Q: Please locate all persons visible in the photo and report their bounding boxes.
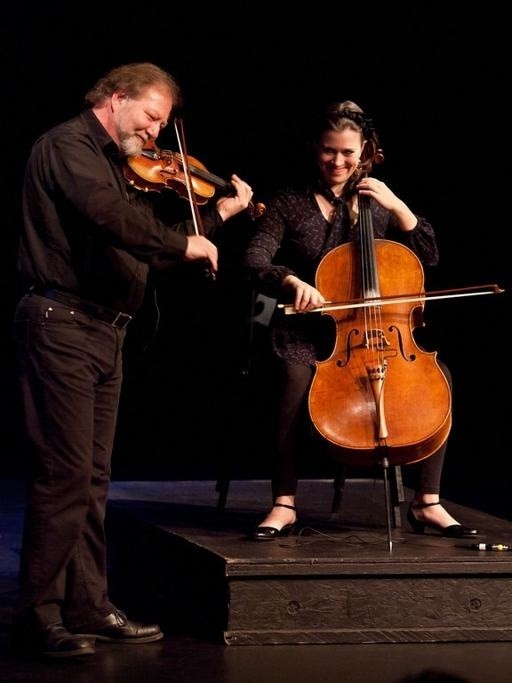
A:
[244,98,483,542]
[13,60,255,661]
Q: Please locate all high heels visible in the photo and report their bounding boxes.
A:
[406,501,479,538]
[253,502,299,540]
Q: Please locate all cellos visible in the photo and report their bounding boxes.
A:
[305,139,454,463]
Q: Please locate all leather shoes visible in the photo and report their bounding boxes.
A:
[42,622,96,658]
[73,608,165,644]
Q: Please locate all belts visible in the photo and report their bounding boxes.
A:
[34,287,134,330]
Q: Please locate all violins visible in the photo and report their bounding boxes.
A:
[123,142,266,219]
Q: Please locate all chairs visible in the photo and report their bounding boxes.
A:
[214,289,405,527]
